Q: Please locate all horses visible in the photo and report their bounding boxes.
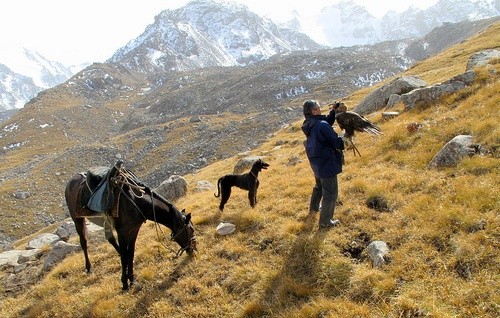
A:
[65,161,197,291]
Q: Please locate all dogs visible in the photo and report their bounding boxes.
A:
[214,159,270,212]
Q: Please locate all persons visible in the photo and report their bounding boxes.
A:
[301,100,353,229]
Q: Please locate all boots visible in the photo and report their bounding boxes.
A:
[310,187,322,212]
[318,196,340,230]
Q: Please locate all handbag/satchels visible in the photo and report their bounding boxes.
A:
[87,174,114,213]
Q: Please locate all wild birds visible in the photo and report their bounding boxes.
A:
[329,101,386,159]
